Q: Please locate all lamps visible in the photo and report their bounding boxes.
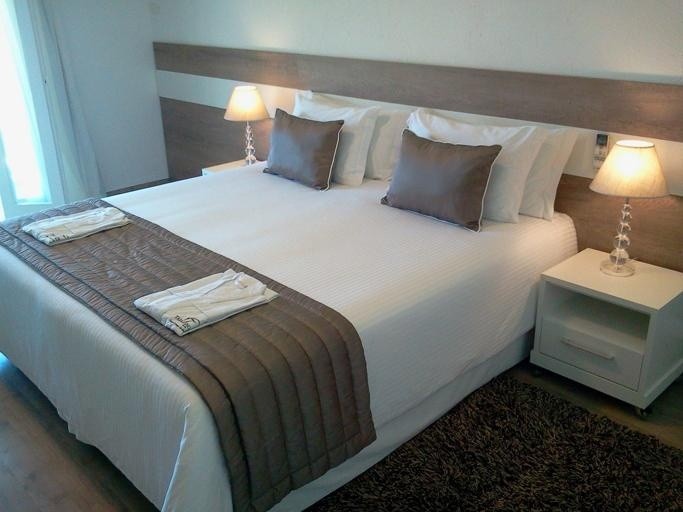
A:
[586,136,672,278]
[223,84,270,165]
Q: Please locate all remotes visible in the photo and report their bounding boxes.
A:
[593,133,609,169]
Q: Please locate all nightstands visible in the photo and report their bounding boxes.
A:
[528,245,683,420]
[201,158,262,174]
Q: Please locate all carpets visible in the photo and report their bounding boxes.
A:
[304,372,682,510]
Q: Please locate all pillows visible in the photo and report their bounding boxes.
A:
[516,123,580,220]
[383,108,549,227]
[302,87,413,182]
[262,107,345,192]
[291,91,381,187]
[381,128,503,234]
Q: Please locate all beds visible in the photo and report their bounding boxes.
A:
[0,158,579,510]
